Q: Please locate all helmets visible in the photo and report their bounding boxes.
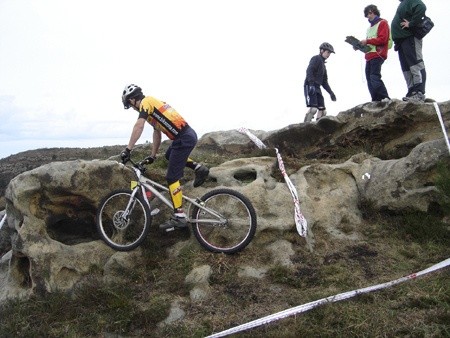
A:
[319,42,335,53]
[122,83,141,109]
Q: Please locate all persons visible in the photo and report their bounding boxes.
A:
[304,42,336,123]
[353,5,393,101]
[120,83,210,227]
[391,0,427,102]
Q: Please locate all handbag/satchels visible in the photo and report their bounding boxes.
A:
[411,14,434,40]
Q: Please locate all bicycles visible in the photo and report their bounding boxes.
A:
[94,152,257,255]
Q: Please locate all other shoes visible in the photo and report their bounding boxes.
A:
[193,165,209,187]
[159,215,188,230]
[402,91,426,102]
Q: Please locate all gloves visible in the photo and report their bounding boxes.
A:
[120,148,131,164]
[144,156,155,164]
[330,93,336,101]
